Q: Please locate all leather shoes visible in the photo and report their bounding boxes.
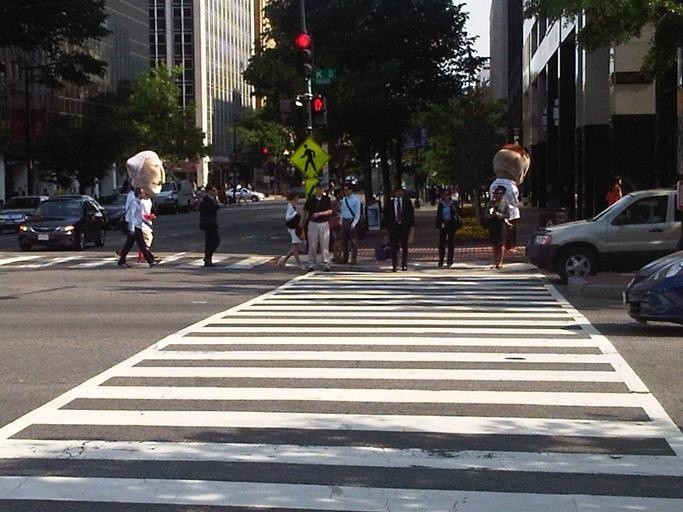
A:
[277,258,503,272]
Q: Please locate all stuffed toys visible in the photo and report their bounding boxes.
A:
[489,143,531,251]
[125,149,166,261]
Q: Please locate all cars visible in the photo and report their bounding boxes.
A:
[0,178,266,250]
[622,249,681,330]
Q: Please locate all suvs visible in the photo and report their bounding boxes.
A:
[527,187,681,285]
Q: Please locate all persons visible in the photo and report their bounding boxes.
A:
[171,183,252,214]
[487,185,510,268]
[278,184,363,269]
[199,186,221,267]
[363,184,483,271]
[17,185,64,196]
[605,173,623,206]
[117,187,162,268]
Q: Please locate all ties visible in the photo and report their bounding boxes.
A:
[395,198,403,226]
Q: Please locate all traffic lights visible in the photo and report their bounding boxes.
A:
[310,94,328,127]
[262,147,274,155]
[292,30,314,78]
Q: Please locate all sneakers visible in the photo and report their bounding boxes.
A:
[118,256,161,270]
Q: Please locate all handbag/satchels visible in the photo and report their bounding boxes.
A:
[116,216,131,235]
[286,212,301,230]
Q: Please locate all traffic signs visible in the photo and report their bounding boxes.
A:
[311,66,337,85]
